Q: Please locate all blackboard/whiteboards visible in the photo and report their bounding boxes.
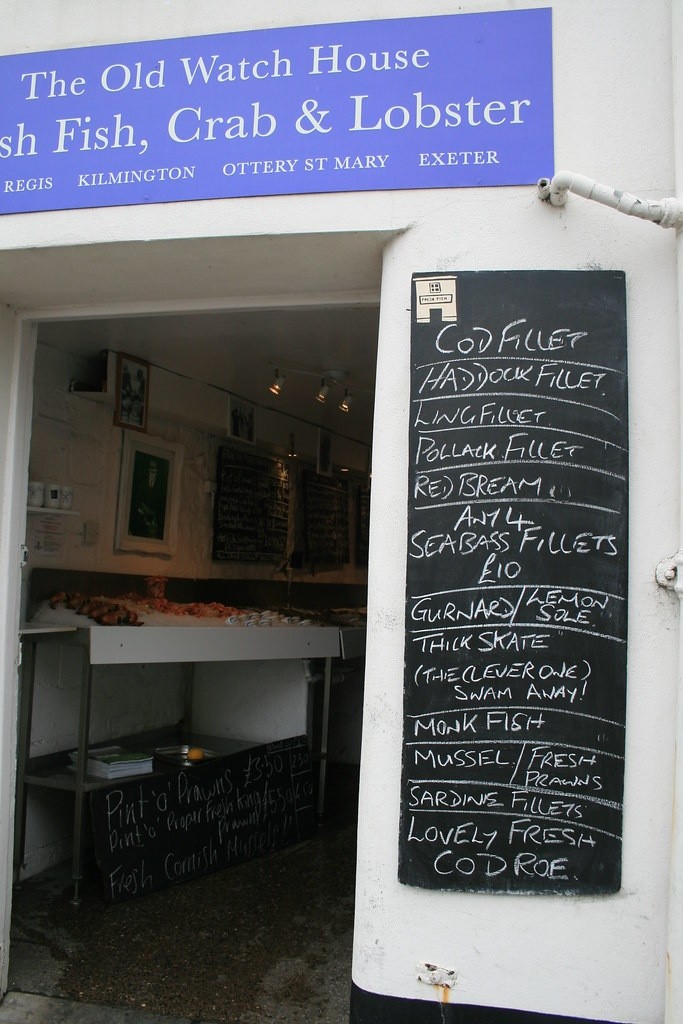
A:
[90,734,317,905]
[355,485,371,569]
[397,268,630,895]
[212,445,289,566]
[302,469,351,565]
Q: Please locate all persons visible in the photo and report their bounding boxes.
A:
[231,407,253,441]
[131,458,163,540]
[120,364,145,401]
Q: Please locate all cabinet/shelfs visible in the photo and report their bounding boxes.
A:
[15,627,333,906]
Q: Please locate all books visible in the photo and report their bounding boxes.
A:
[70,746,154,780]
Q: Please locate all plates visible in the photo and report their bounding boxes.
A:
[152,743,221,766]
[69,745,154,780]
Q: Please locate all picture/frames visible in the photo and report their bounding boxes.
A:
[317,427,334,477]
[227,396,257,444]
[115,428,185,556]
[113,352,150,434]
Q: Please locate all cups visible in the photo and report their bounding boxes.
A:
[28,481,45,507]
[42,484,60,509]
[59,486,73,510]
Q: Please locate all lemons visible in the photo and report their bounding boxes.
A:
[188,749,203,759]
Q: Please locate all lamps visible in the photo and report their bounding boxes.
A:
[269,370,289,395]
[320,369,346,382]
[315,377,330,403]
[338,388,352,412]
